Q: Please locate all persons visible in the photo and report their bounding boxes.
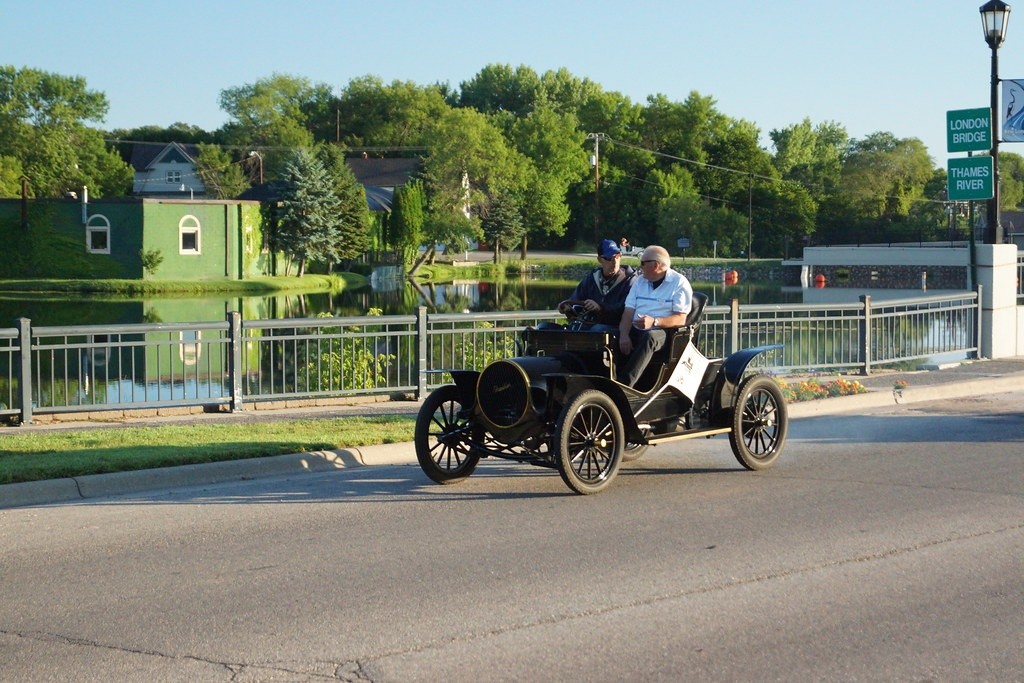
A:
[622,238,632,252]
[589,246,693,388]
[535,240,636,331]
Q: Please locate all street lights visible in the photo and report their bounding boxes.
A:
[980,0,1013,244]
[248,150,264,186]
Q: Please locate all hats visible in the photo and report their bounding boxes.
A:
[597,239,620,257]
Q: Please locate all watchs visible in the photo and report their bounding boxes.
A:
[654,317,659,327]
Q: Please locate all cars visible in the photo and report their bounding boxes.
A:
[413,289,790,497]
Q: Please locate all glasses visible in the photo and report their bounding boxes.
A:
[600,253,620,260]
[641,259,660,266]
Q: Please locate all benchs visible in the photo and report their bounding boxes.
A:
[651,291,709,363]
